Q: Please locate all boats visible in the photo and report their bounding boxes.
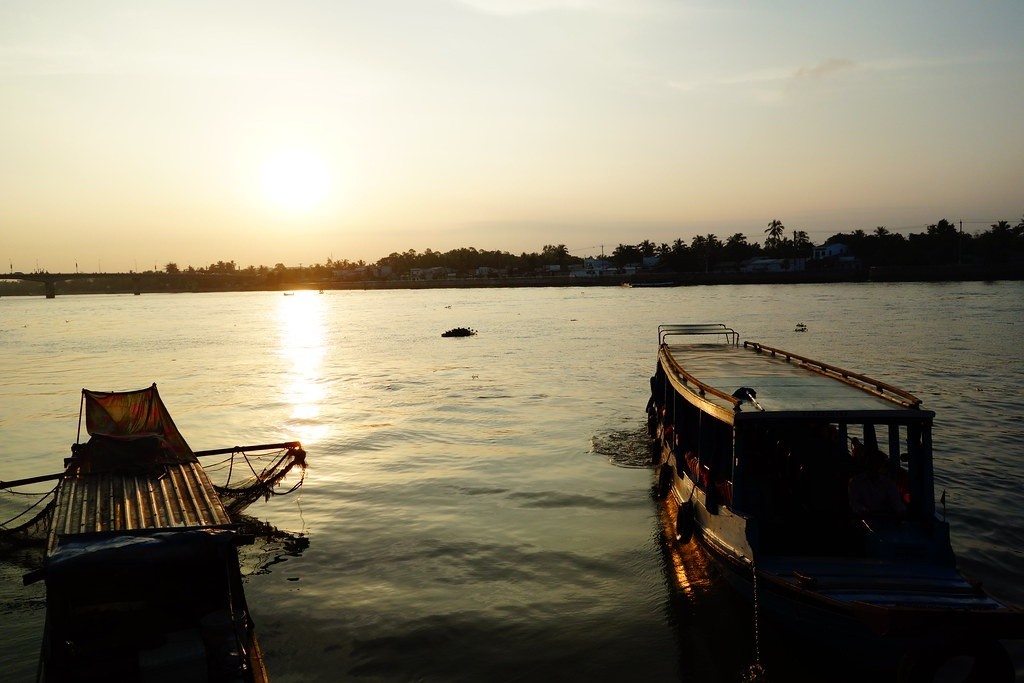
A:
[33,434,270,683]
[647,323,1024,671]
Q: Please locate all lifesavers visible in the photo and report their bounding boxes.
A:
[652,438,661,465]
[675,502,694,544]
[648,414,656,436]
[659,462,669,497]
